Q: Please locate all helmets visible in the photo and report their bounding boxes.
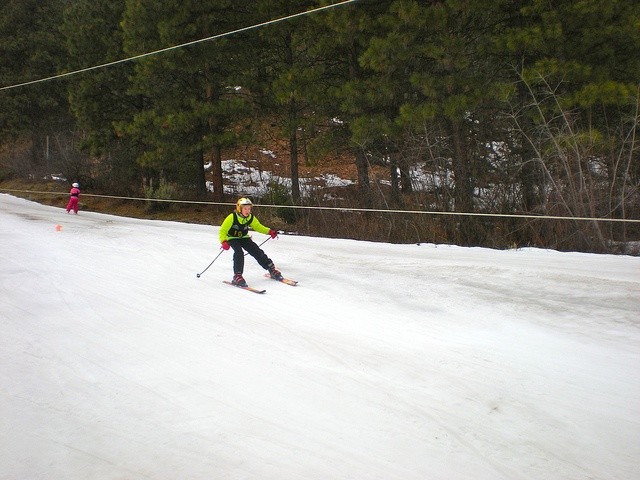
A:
[72,183,79,188]
[236,197,253,213]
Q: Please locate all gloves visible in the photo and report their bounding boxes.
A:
[222,240,230,250]
[269,229,277,239]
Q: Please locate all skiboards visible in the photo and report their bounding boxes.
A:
[222,272,299,294]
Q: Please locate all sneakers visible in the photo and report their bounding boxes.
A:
[232,272,248,287]
[269,264,284,281]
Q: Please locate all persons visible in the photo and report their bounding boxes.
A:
[218,197,282,285]
[65,183,81,215]
